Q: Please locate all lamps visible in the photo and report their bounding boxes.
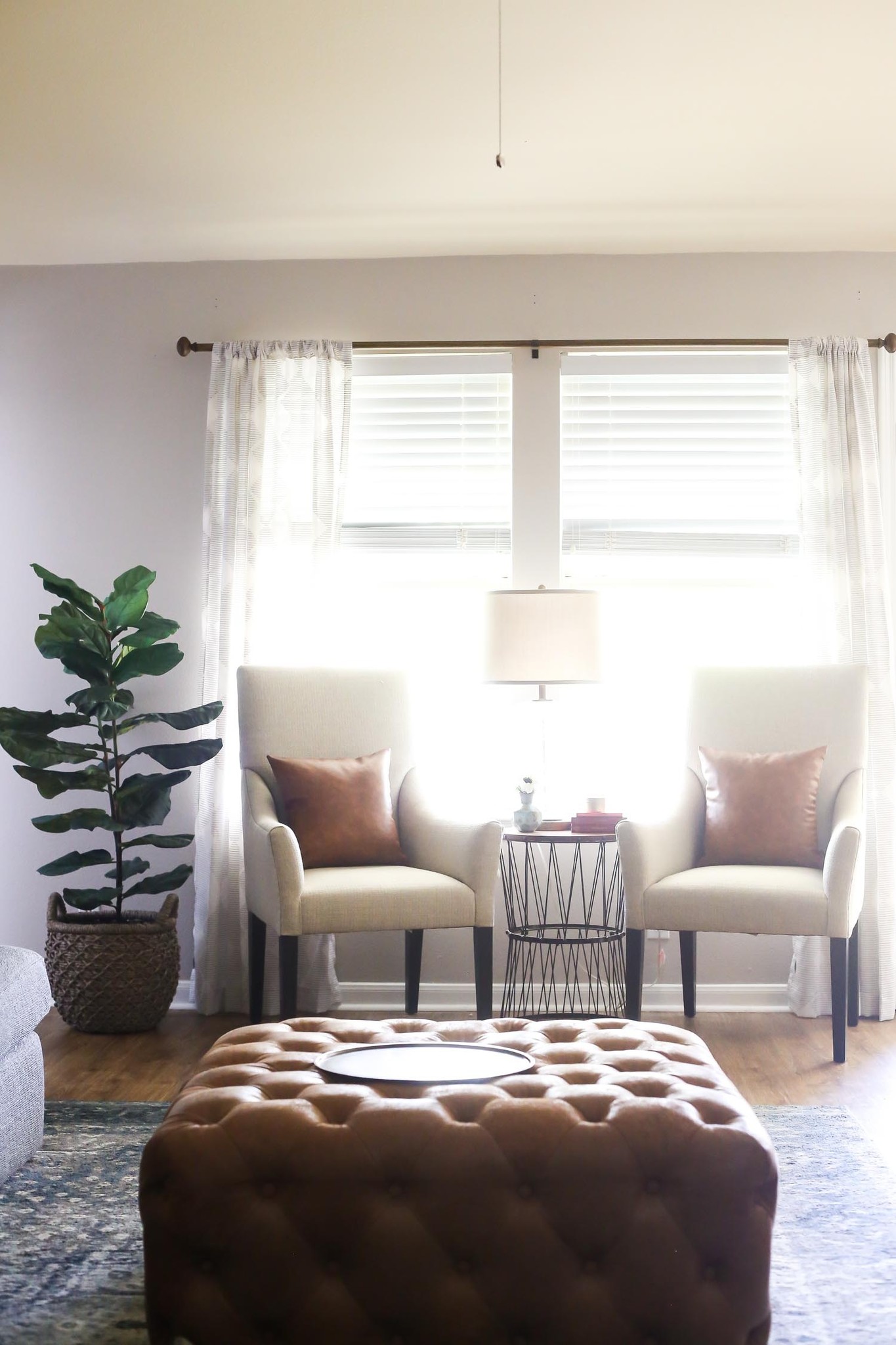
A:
[487,585,601,830]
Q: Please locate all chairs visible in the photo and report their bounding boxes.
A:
[615,661,865,1063]
[237,666,504,1025]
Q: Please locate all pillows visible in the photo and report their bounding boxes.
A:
[697,746,828,870]
[266,749,409,869]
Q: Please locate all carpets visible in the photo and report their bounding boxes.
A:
[0,1100,896,1345]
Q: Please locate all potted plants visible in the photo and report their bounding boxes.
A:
[0,564,223,1032]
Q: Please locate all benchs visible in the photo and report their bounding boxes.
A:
[138,1016,778,1345]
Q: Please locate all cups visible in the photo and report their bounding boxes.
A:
[587,798,605,812]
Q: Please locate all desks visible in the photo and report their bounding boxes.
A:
[500,827,626,1018]
